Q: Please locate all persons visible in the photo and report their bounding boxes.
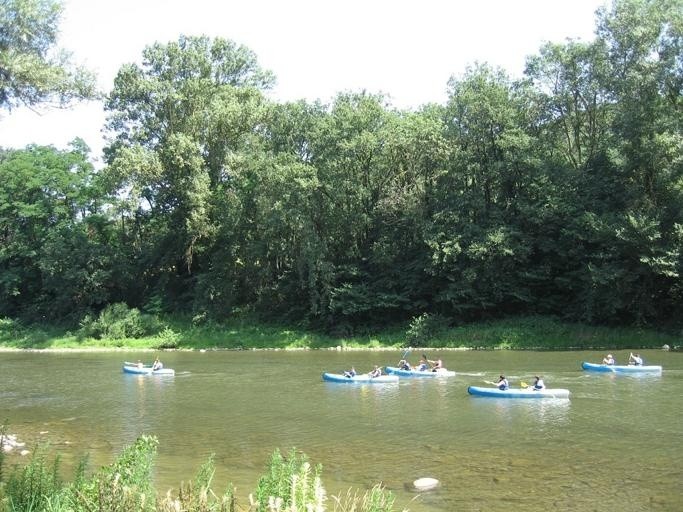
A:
[491,375,508,391]
[398,360,411,371]
[344,367,356,378]
[368,366,381,378]
[133,360,143,369]
[431,362,441,372]
[604,354,614,366]
[152,360,162,371]
[530,376,545,391]
[631,352,642,366]
[416,354,428,372]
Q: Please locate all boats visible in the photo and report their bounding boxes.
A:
[468,386,569,397]
[582,363,662,373]
[123,366,175,375]
[322,367,455,383]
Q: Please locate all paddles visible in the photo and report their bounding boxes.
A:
[485,381,497,385]
[125,361,147,366]
[423,354,437,372]
[147,356,159,375]
[521,382,541,390]
